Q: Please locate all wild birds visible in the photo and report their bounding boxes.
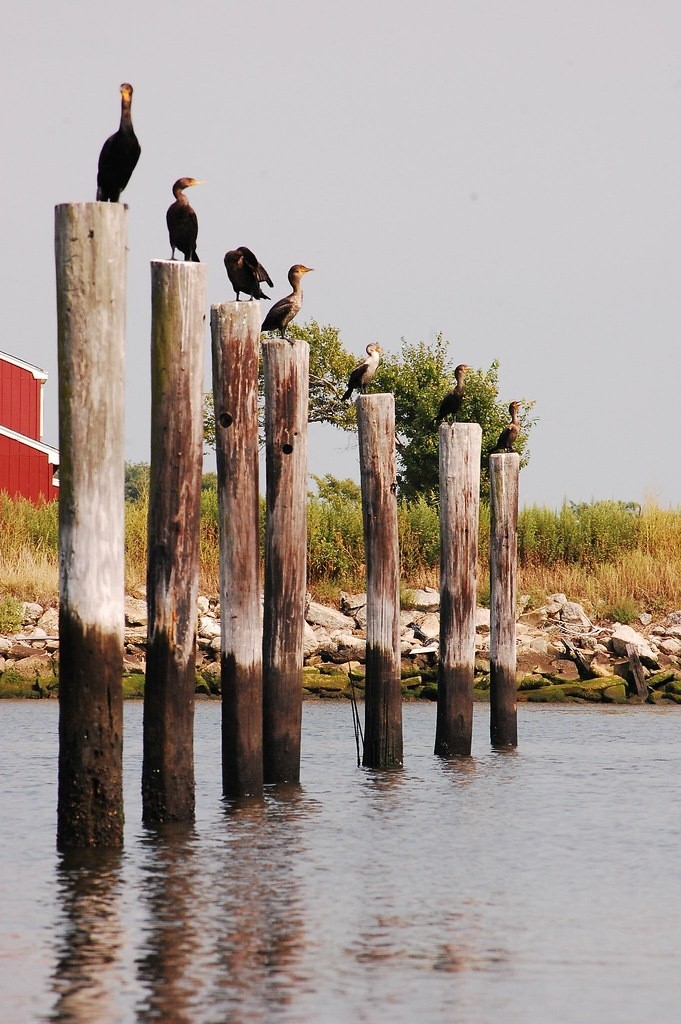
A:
[339,340,383,403]
[487,399,526,454]
[224,243,275,303]
[94,84,143,205]
[430,363,473,434]
[261,263,315,345]
[164,177,207,263]
